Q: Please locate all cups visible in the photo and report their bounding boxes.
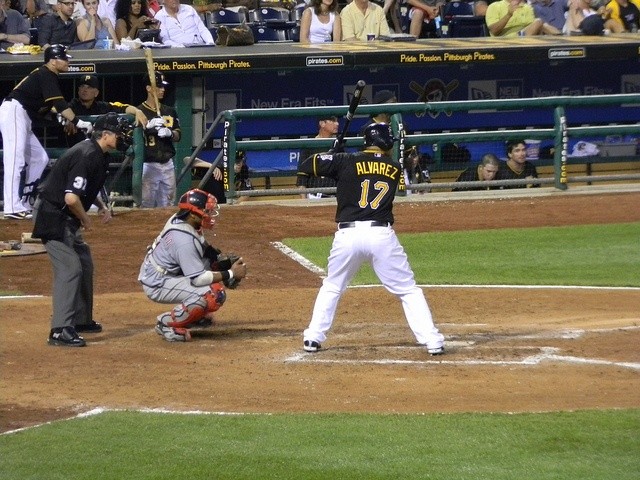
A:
[102,39,114,50]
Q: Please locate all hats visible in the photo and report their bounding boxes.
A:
[142,71,168,88]
[371,90,394,103]
[78,75,99,88]
[44,44,73,58]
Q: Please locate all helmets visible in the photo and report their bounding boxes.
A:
[174,188,220,230]
[92,112,134,152]
[365,122,394,151]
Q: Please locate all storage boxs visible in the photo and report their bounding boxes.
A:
[596,138,638,157]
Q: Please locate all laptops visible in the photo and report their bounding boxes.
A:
[379,32,416,42]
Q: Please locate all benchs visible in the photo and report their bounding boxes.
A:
[191,134,639,184]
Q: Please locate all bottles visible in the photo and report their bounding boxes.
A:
[323,31,331,42]
[192,34,199,43]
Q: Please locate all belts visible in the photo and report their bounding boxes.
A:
[338,220,389,228]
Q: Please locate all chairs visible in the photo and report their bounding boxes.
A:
[248,7,291,41]
[204,7,249,42]
[293,5,308,39]
[442,1,487,38]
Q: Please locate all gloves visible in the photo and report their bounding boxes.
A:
[56,112,69,126]
[77,118,95,134]
[146,118,166,127]
[157,127,172,138]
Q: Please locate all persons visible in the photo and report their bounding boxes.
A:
[64,68,146,208]
[396,0,445,39]
[126,70,183,208]
[297,0,344,45]
[185,2,222,14]
[564,0,606,36]
[152,0,217,47]
[484,0,557,37]
[136,188,248,342]
[361,90,434,196]
[336,0,395,41]
[0,44,90,220]
[533,0,574,36]
[36,0,78,45]
[0,0,33,50]
[76,0,120,48]
[30,110,131,348]
[299,120,448,357]
[498,139,539,190]
[114,0,162,50]
[292,116,349,200]
[602,0,639,35]
[450,152,503,190]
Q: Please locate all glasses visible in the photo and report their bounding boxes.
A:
[57,0,75,5]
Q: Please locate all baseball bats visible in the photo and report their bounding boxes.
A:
[143,47,162,116]
[320,80,368,181]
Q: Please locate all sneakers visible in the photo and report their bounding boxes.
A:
[46,327,85,346]
[75,320,102,333]
[154,321,191,342]
[427,347,446,355]
[302,340,321,352]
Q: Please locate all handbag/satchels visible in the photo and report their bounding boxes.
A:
[30,207,66,243]
[217,21,256,47]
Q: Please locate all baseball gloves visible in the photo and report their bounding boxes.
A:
[219,253,246,288]
